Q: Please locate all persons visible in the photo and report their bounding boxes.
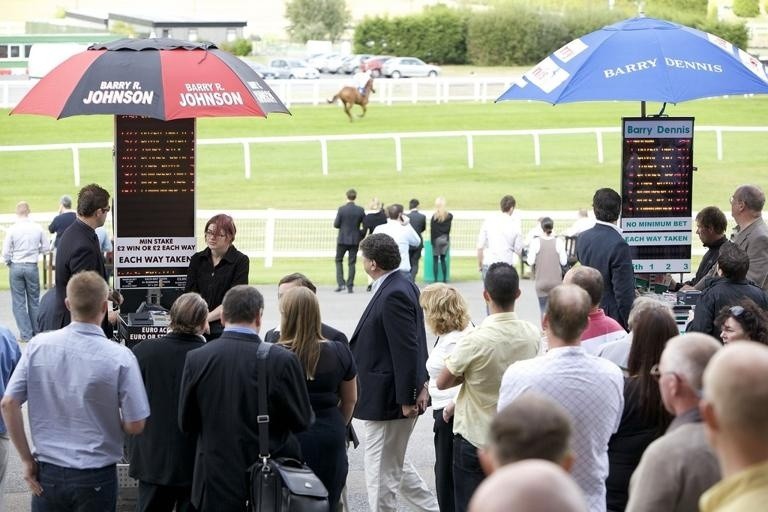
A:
[1,186,438,511]
[418,186,767,512]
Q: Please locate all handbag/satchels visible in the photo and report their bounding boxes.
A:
[249,457,330,512]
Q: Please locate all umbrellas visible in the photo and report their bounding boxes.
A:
[8,33,295,122]
[489,16,767,116]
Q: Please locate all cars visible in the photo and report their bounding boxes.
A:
[234,49,442,81]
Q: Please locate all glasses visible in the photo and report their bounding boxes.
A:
[205,230,229,238]
[651,366,676,380]
[102,205,111,213]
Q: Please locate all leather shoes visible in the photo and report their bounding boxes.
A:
[334,286,345,292]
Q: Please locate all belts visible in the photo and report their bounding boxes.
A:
[12,261,38,267]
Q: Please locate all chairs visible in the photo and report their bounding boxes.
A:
[564,235,577,258]
[521,236,558,279]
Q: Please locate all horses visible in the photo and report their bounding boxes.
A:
[326,78,376,119]
